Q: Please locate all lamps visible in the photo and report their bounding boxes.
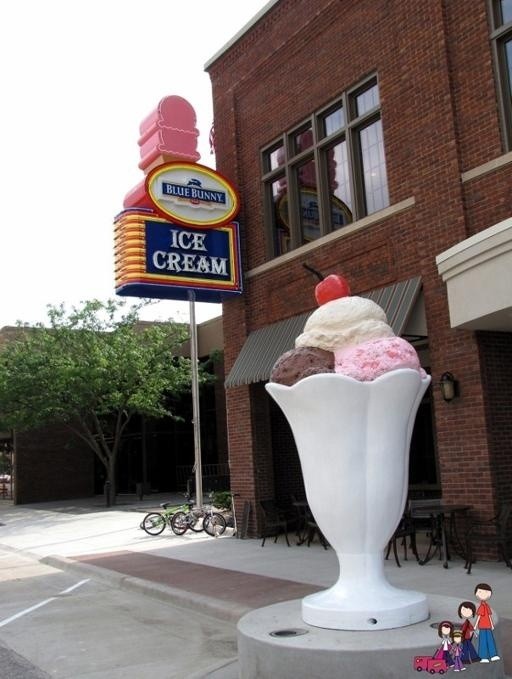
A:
[434,370,461,402]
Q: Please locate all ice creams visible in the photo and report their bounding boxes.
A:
[268,273,428,385]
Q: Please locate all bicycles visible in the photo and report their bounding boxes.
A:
[141,488,230,537]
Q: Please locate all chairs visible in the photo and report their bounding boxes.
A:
[258,492,512,575]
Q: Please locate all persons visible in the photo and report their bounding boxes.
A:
[436,583,500,670]
